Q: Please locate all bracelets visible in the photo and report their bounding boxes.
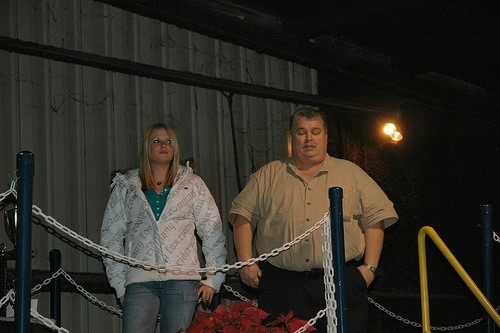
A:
[363,262,379,276]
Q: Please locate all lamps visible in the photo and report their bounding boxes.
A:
[382,121,403,142]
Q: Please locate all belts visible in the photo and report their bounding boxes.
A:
[264,253,365,277]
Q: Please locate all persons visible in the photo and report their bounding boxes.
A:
[100,122,227,333]
[226,106,400,333]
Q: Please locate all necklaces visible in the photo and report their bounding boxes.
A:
[153,175,166,185]
